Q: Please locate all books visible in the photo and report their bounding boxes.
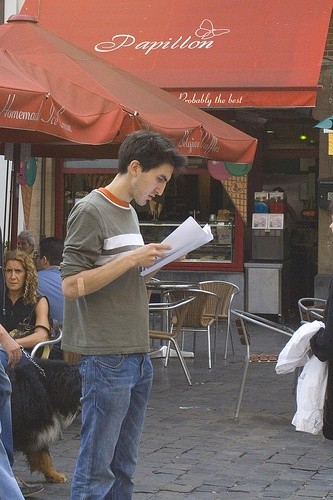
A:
[140,216,214,276]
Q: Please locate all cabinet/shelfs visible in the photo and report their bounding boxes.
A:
[138,222,233,263]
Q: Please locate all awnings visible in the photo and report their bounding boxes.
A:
[19,0,333,108]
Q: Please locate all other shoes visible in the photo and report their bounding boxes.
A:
[15,476,44,496]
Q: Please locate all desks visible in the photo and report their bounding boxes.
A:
[145,281,198,359]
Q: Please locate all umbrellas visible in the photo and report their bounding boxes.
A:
[0,15,258,250]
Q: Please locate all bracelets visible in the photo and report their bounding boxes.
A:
[34,325,49,332]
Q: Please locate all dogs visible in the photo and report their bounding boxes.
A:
[4,358,82,483]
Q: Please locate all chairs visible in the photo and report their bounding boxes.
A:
[298,298,326,324]
[48,319,80,365]
[29,329,64,440]
[230,309,301,422]
[192,280,241,360]
[148,296,196,386]
[161,289,222,370]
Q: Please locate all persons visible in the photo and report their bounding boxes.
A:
[0,251,67,483]
[35,236,64,325]
[311,201,333,441]
[0,323,44,500]
[60,129,184,500]
[19,232,36,255]
